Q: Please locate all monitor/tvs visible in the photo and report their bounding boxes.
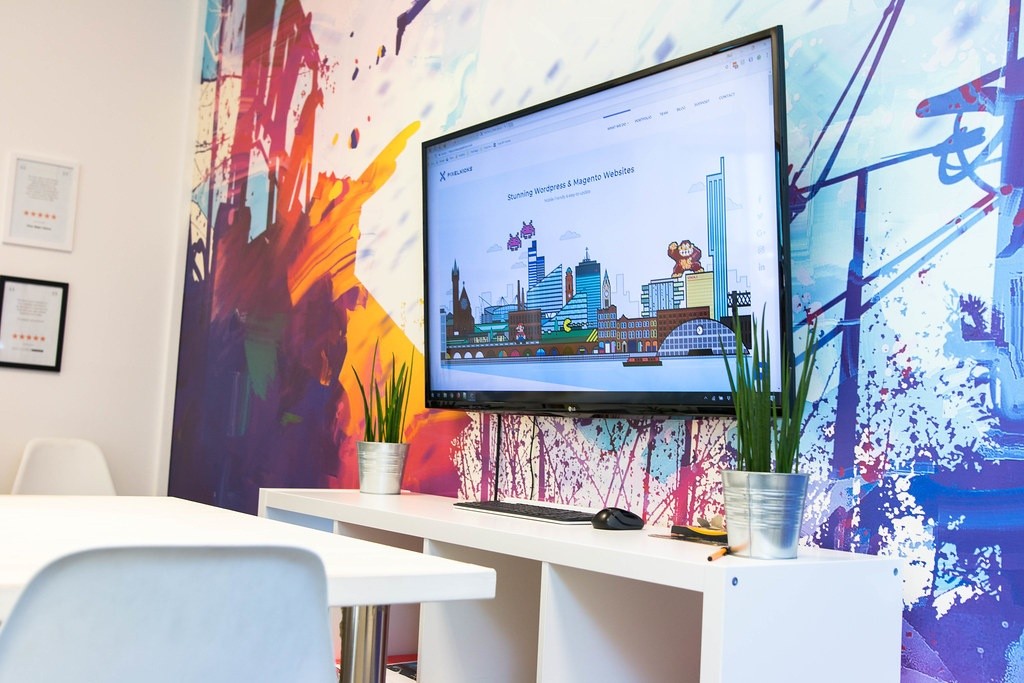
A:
[422,25,795,417]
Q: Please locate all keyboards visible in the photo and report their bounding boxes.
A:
[453,500,596,524]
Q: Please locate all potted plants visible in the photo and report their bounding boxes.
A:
[351,337,416,495]
[720,297,839,563]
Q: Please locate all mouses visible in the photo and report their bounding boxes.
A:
[592,506,645,530]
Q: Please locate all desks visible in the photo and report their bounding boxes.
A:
[0,493,498,683]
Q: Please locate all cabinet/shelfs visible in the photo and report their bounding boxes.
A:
[259,488,907,683]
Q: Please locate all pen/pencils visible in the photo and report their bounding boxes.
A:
[706,545,731,561]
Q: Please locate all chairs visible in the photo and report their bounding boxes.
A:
[0,543,340,683]
[8,436,116,496]
